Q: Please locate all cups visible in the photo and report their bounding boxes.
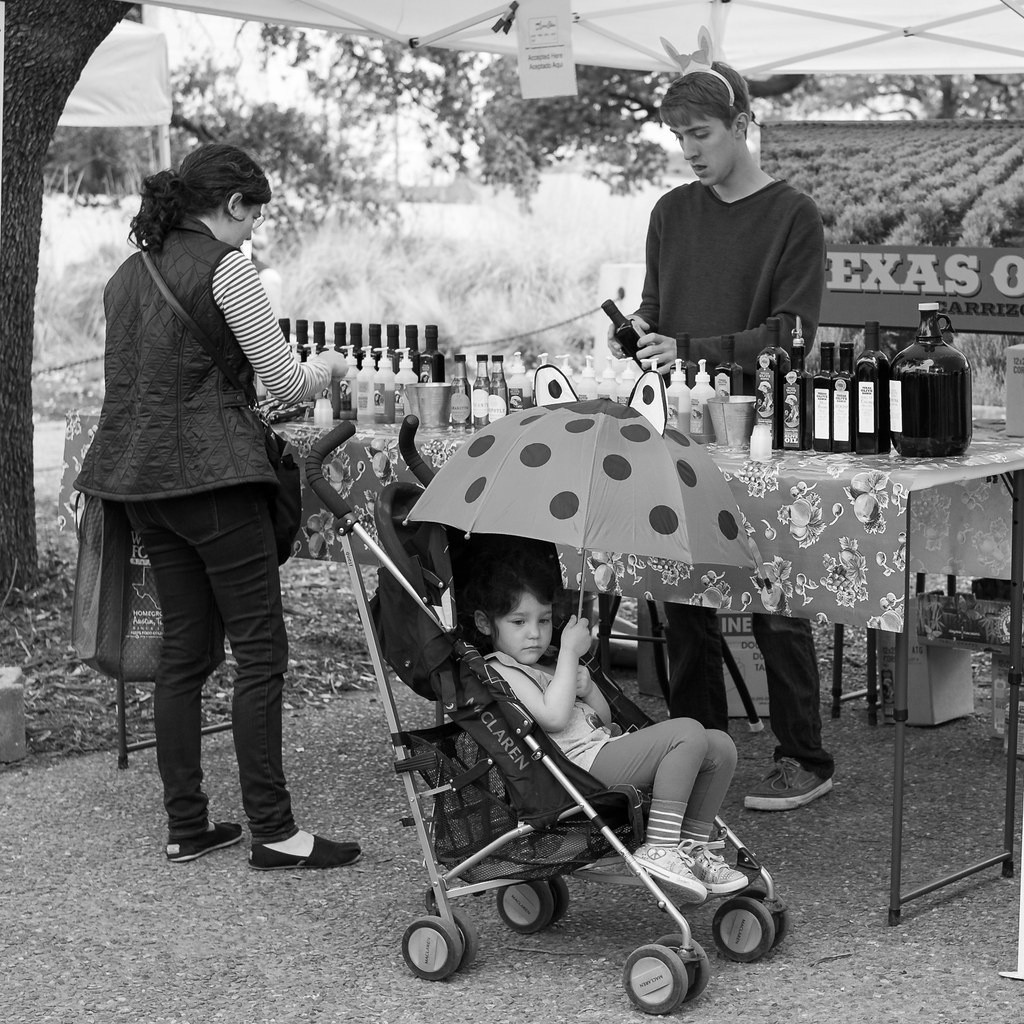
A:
[313,399,334,425]
[750,425,773,461]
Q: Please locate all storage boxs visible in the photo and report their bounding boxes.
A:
[636,594,770,719]
[874,595,1024,755]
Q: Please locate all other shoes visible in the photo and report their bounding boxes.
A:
[249,833,362,868]
[166,820,244,863]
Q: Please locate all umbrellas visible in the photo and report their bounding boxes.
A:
[402,364,757,620]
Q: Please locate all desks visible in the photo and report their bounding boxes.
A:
[58,412,1024,926]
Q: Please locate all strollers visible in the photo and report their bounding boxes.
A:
[304,415,789,1015]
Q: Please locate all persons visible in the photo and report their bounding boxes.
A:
[72,145,362,870]
[606,61,834,810]
[463,556,748,904]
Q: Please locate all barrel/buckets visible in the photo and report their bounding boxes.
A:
[405,382,453,428]
[706,395,756,447]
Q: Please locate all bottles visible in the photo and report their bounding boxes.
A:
[670,333,698,389]
[755,317,791,449]
[450,354,472,430]
[813,341,838,453]
[279,318,444,383]
[714,335,744,395]
[831,342,857,453]
[473,354,490,430]
[488,354,508,425]
[890,302,972,457]
[782,338,813,452]
[601,299,671,389]
[1002,676,1024,760]
[856,320,890,455]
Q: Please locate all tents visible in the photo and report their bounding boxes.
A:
[58,19,174,171]
[116,0,1024,76]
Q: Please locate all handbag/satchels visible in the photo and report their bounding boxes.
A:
[873,594,974,725]
[71,497,226,682]
[256,414,303,565]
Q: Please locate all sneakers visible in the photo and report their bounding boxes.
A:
[745,756,831,809]
[683,839,751,893]
[634,838,707,903]
